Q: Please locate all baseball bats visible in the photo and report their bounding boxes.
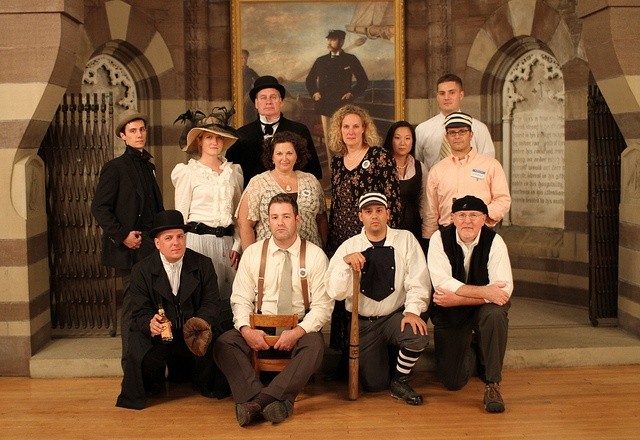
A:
[348,264,362,401]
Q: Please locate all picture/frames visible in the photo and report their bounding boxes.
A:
[225,1,410,213]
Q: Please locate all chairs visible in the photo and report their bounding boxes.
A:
[249,313,299,380]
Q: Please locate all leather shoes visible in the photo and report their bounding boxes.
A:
[263,399,293,422]
[235,402,261,426]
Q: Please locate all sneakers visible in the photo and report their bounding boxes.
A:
[484,384,505,411]
[390,380,423,406]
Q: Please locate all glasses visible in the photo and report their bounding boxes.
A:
[455,214,485,220]
[446,130,471,136]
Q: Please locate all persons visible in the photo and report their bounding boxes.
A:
[236,131,330,271]
[89,109,168,380]
[323,191,432,408]
[240,46,263,104]
[413,68,496,183]
[305,27,370,159]
[166,113,246,344]
[115,207,228,410]
[324,104,402,251]
[427,193,515,414]
[426,113,512,241]
[227,74,317,191]
[221,191,333,426]
[381,120,431,239]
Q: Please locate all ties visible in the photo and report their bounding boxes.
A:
[441,136,451,160]
[275,251,293,335]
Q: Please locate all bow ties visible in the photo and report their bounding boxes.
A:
[258,119,278,135]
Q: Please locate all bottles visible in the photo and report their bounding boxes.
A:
[158,303,173,342]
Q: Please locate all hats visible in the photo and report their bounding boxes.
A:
[452,194,488,212]
[443,112,473,129]
[325,30,345,39]
[145,210,192,239]
[114,109,148,136]
[248,76,285,103]
[174,106,239,154]
[358,192,388,210]
